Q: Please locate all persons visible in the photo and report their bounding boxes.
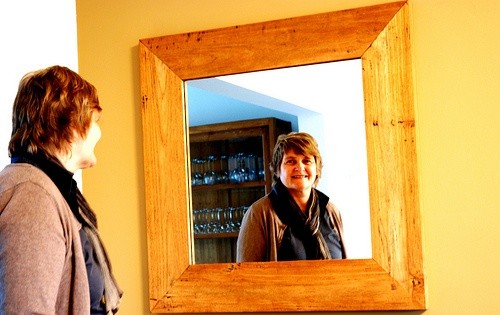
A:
[236,132,348,263]
[0,65,126,315]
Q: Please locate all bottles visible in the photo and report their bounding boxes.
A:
[258,158,264,180]
[193,206,248,234]
[191,153,255,185]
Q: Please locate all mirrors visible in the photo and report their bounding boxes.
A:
[140,1,429,315]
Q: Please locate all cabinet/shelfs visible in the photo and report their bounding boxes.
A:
[189,117,293,264]
